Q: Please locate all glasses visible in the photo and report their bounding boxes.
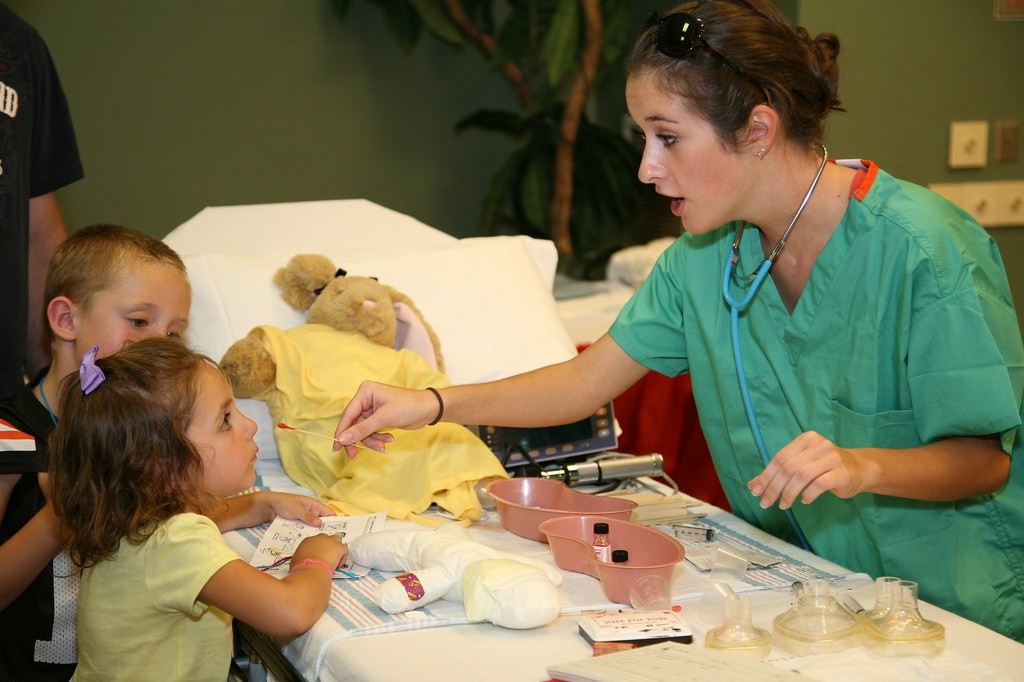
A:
[638,11,777,109]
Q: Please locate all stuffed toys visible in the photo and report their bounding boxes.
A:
[220,252,509,524]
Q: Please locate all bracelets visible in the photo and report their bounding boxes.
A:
[423,386,443,426]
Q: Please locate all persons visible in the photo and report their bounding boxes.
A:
[2,224,191,681]
[333,1,1024,646]
[1,0,85,408]
[48,338,348,682]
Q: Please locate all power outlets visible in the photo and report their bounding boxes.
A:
[995,118,1021,166]
